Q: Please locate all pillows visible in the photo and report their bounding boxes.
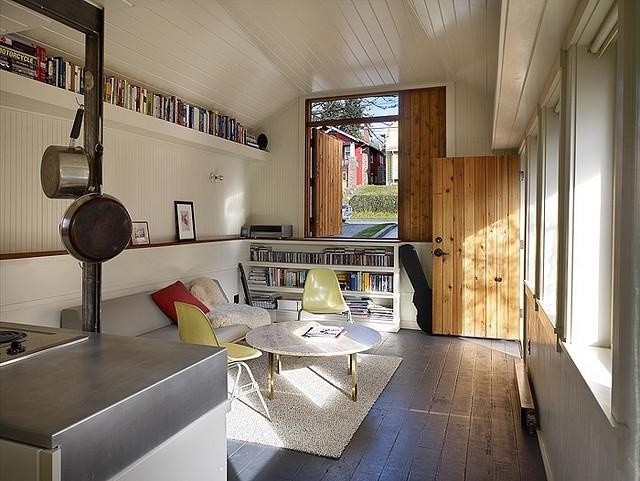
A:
[148,279,211,328]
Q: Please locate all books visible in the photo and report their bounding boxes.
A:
[247,241,395,319]
[0,33,258,147]
[302,323,347,342]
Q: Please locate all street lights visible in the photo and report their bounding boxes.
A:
[345,158,349,188]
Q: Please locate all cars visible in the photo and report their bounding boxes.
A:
[342,204,353,223]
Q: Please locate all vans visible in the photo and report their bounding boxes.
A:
[388,178,398,185]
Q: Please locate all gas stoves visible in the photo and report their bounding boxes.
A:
[0,322,90,372]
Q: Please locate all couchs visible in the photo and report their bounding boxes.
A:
[58,277,267,352]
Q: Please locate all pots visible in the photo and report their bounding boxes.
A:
[59,142,134,262]
[41,105,92,198]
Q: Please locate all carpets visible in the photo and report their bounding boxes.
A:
[227,351,405,463]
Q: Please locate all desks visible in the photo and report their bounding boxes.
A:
[244,319,385,404]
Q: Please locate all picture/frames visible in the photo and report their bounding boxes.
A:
[128,220,153,248]
[174,198,199,245]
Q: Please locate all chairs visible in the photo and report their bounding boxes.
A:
[171,299,274,428]
[297,267,354,327]
[237,262,279,313]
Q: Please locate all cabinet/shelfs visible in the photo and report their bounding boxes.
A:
[249,241,399,334]
